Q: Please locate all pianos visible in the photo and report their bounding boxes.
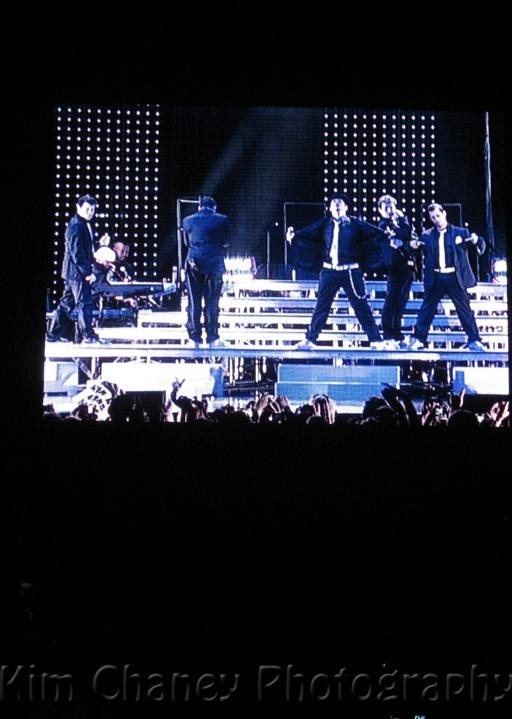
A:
[90,279,176,298]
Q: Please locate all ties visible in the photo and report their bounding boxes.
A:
[329,219,341,266]
[438,229,447,269]
[86,222,94,252]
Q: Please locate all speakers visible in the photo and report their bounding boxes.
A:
[126,391,166,419]
[421,203,463,227]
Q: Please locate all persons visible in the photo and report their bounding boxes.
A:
[46,194,109,346]
[106,239,140,330]
[284,191,398,348]
[401,203,490,352]
[180,195,232,347]
[373,193,422,348]
[37,370,512,447]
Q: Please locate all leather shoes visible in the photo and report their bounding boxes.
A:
[370,337,424,351]
[186,338,229,348]
[294,338,315,349]
[48,333,70,343]
[80,337,111,346]
[467,340,487,352]
